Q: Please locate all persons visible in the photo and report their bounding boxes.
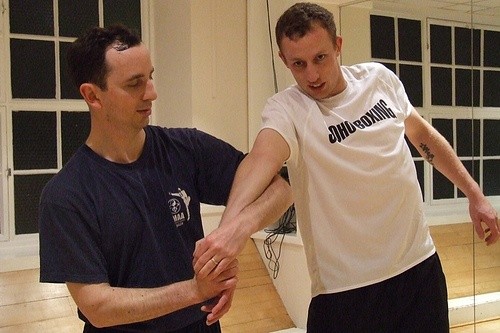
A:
[207,2,500,333]
[39,24,295,333]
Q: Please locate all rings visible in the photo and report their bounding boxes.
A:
[211,259,218,265]
